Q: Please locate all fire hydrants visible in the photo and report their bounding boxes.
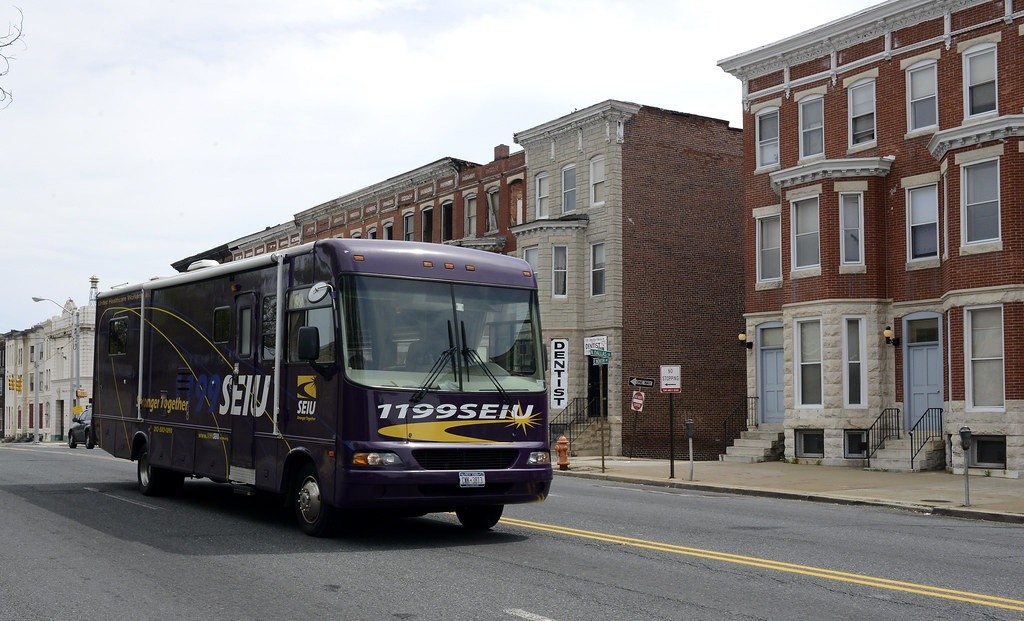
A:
[554,434,571,470]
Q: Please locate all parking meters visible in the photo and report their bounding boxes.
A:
[959,425,972,506]
[684,417,696,482]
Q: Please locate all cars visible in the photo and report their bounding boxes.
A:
[68,408,92,449]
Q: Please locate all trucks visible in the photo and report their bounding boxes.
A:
[88,237,553,536]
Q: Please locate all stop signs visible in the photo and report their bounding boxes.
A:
[631,391,645,411]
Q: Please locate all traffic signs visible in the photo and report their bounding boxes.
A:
[628,377,655,388]
[659,365,681,392]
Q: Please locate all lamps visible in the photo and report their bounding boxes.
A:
[884,326,900,347]
[738,332,752,349]
